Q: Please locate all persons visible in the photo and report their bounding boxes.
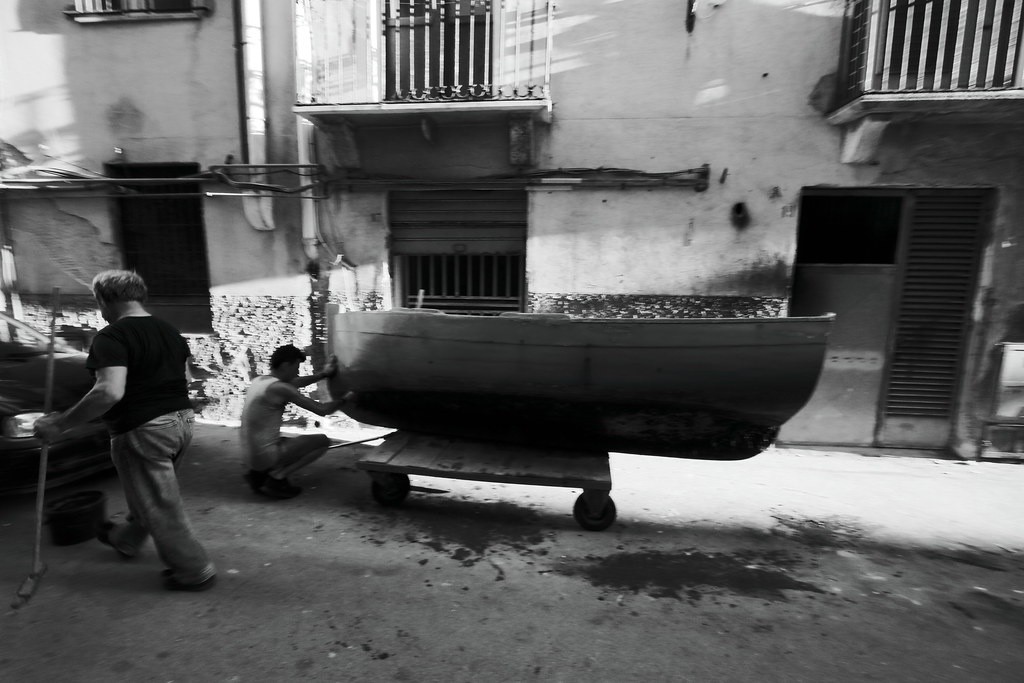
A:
[34,269,217,592]
[233,342,356,500]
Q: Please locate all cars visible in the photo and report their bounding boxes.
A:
[0,311,113,496]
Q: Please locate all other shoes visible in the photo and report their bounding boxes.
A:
[165,572,218,592]
[96,521,135,561]
[260,485,303,498]
[242,473,265,495]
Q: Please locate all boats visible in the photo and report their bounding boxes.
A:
[326,301,837,461]
